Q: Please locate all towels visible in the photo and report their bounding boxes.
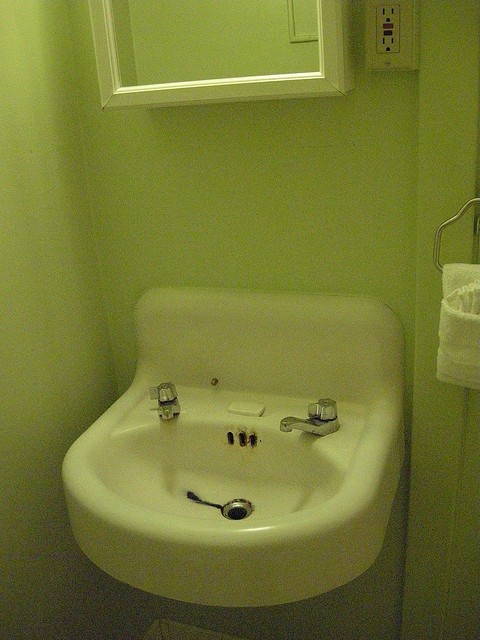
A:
[434,263,480,390]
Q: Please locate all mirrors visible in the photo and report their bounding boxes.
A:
[89,0,355,110]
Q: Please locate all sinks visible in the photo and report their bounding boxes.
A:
[89,414,344,526]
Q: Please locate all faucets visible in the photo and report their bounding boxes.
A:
[150,382,181,421]
[278,398,339,436]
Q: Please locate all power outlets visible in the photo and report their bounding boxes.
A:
[361,0,419,74]
